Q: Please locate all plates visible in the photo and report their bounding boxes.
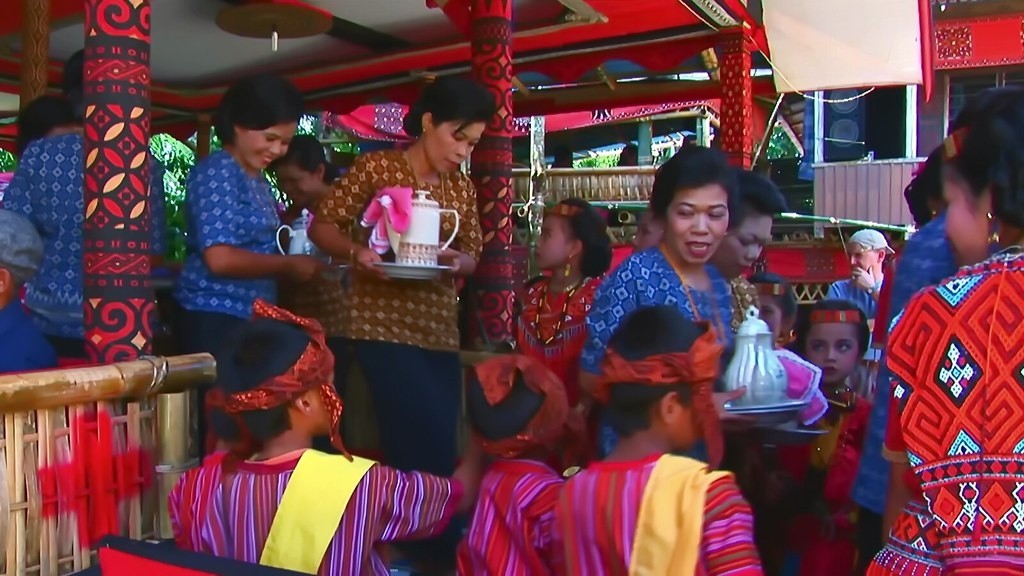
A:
[723,402,829,443]
[373,264,453,280]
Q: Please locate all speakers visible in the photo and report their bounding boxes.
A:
[823,84,906,161]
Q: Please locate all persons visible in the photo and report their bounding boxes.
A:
[0,61,1024,576]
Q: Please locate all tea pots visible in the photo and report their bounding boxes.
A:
[721,304,788,405]
[377,187,459,266]
[276,208,332,267]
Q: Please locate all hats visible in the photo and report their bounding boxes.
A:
[847,229,895,254]
[0,209,45,283]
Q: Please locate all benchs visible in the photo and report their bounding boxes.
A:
[97,534,310,576]
